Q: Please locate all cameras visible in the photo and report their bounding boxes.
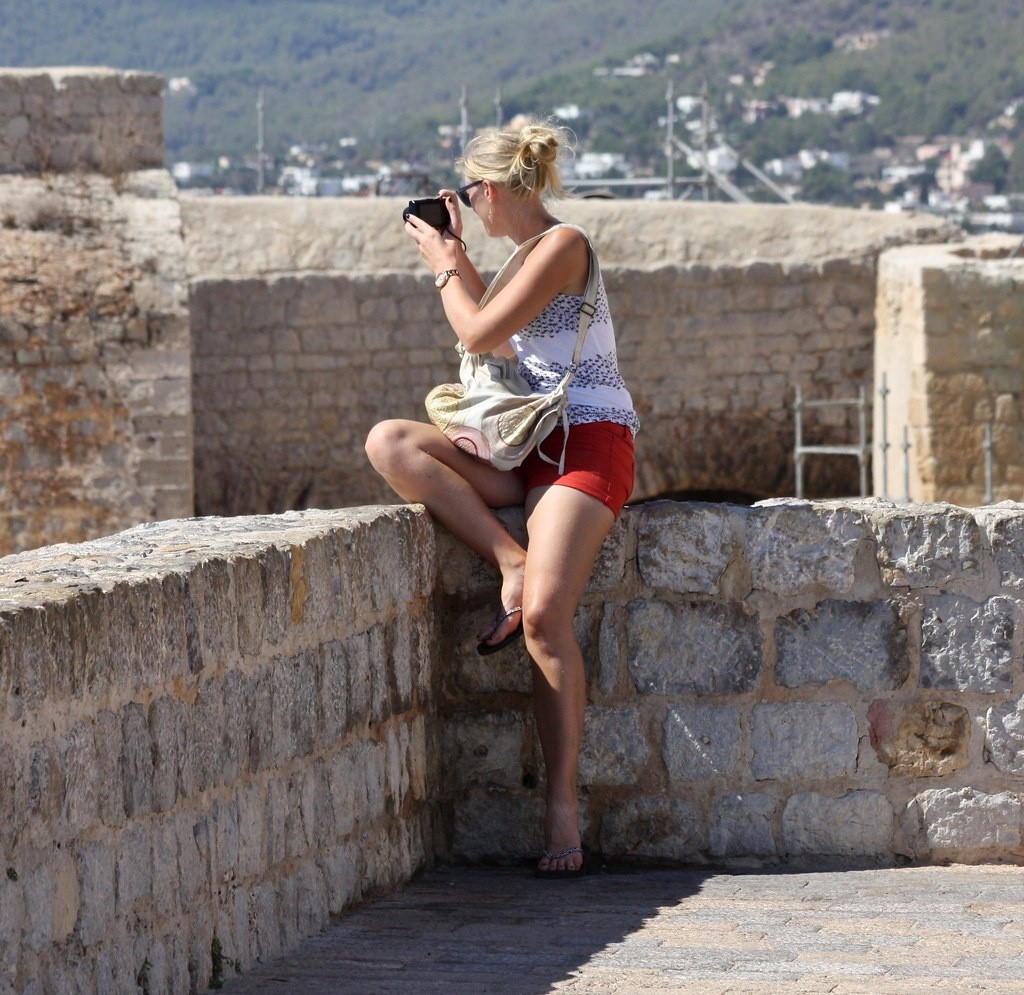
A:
[403,198,452,230]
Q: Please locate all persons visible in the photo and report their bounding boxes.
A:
[365,116,640,879]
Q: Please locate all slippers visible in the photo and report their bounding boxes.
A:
[476,605,522,655]
[537,847,583,877]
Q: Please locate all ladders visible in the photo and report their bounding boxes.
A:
[794,382,869,498]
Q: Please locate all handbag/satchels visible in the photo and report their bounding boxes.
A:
[424,342,570,475]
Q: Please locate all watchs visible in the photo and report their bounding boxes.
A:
[434,269,462,294]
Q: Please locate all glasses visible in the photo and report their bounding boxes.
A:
[455,180,484,209]
